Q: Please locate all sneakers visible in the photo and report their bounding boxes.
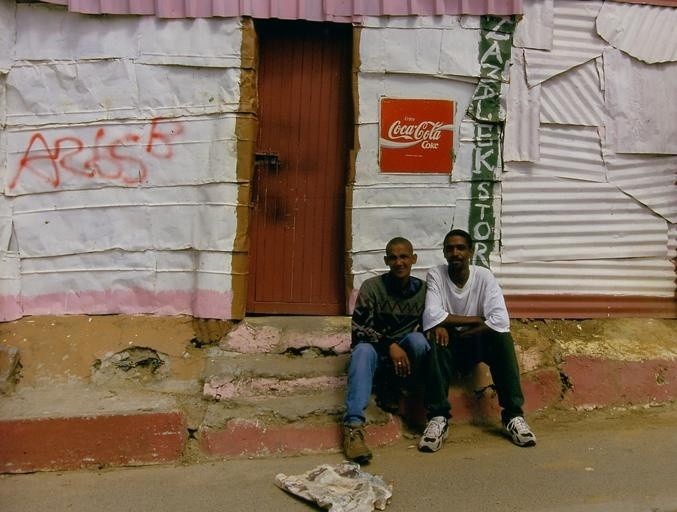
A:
[418,416,449,454]
[500,408,538,448]
[343,422,373,464]
[377,389,401,412]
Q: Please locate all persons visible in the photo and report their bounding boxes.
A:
[419,229,536,452]
[344,237,449,463]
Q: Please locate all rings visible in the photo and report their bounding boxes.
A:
[398,361,401,364]
